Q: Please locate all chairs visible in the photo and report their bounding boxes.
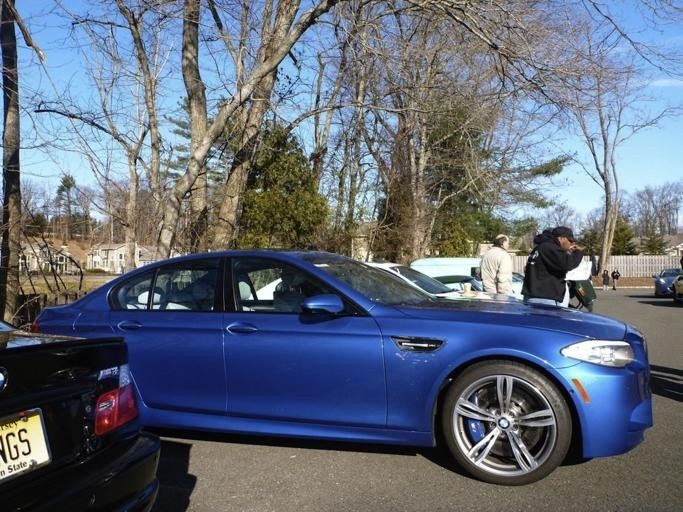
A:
[192,278,255,313]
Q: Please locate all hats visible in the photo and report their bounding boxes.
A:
[552,226,577,242]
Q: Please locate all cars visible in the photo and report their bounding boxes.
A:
[1,319,164,511]
[239,255,531,312]
[651,267,683,297]
[18,241,656,489]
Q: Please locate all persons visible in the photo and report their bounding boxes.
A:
[480,232,512,295]
[610,267,620,290]
[473,268,483,292]
[521,225,586,308]
[601,269,608,291]
